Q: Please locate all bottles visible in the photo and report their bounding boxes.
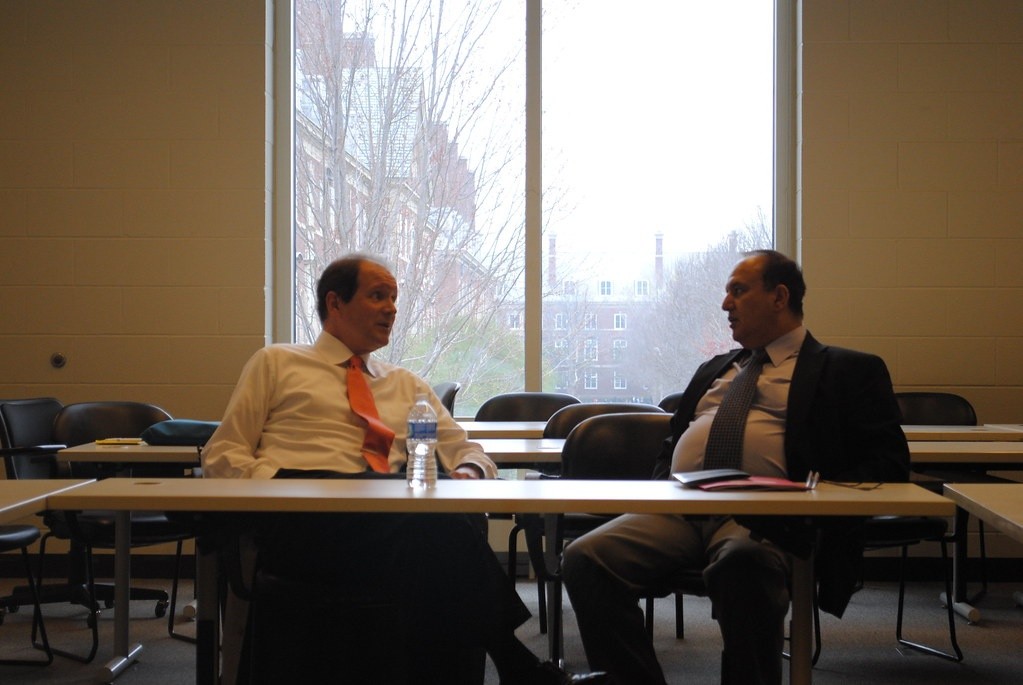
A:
[406,393,440,490]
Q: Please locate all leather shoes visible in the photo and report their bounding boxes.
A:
[499,659,610,685]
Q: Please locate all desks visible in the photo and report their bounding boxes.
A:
[0,479,97,525]
[900,423,1023,625]
[57,438,568,681]
[46,477,958,685]
[184,422,546,621]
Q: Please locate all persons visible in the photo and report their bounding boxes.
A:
[561,250,911,685]
[200,257,607,685]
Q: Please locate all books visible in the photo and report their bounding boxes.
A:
[671,469,811,492]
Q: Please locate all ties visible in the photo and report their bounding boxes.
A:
[703,348,770,469]
[345,356,395,473]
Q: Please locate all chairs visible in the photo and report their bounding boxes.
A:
[0,382,987,668]
